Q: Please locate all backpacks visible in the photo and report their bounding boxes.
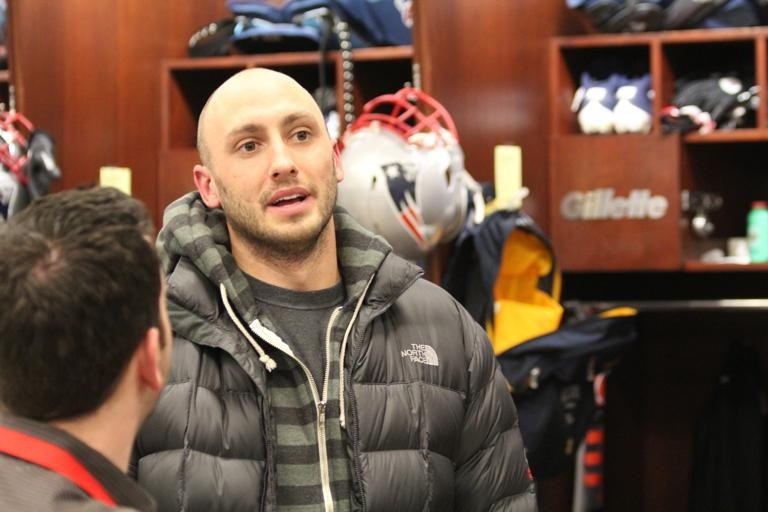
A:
[443,183,639,480]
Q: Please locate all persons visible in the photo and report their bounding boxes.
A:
[126,63,538,510]
[0,186,172,511]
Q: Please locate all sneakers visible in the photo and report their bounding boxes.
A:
[574,73,653,133]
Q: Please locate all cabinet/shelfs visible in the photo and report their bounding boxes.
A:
[155,0,438,285]
[547,25,768,272]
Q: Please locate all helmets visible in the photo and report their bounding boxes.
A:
[332,89,470,258]
[1,111,60,221]
